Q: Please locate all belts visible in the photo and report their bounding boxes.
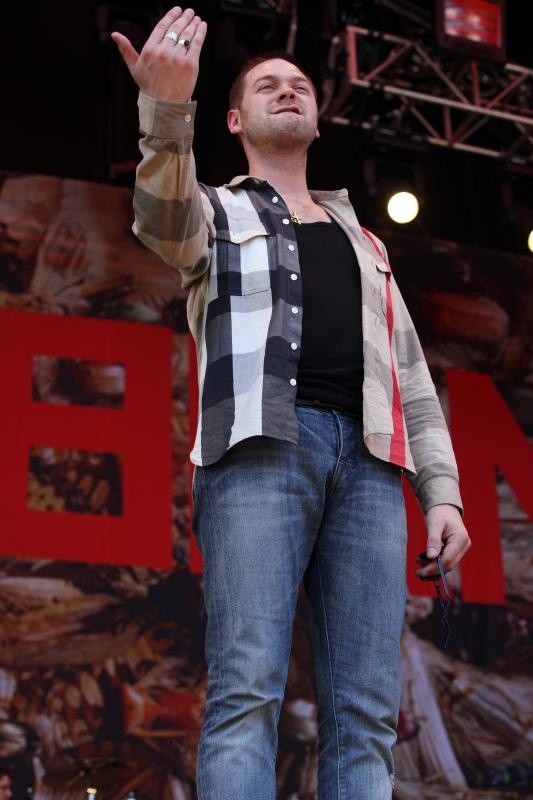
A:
[294,395,359,419]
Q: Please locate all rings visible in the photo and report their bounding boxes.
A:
[178,39,190,47]
[165,32,178,42]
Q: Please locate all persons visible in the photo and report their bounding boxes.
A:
[111,7,471,800]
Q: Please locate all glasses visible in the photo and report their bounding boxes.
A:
[419,551,451,649]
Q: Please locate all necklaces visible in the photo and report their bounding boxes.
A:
[290,194,311,224]
